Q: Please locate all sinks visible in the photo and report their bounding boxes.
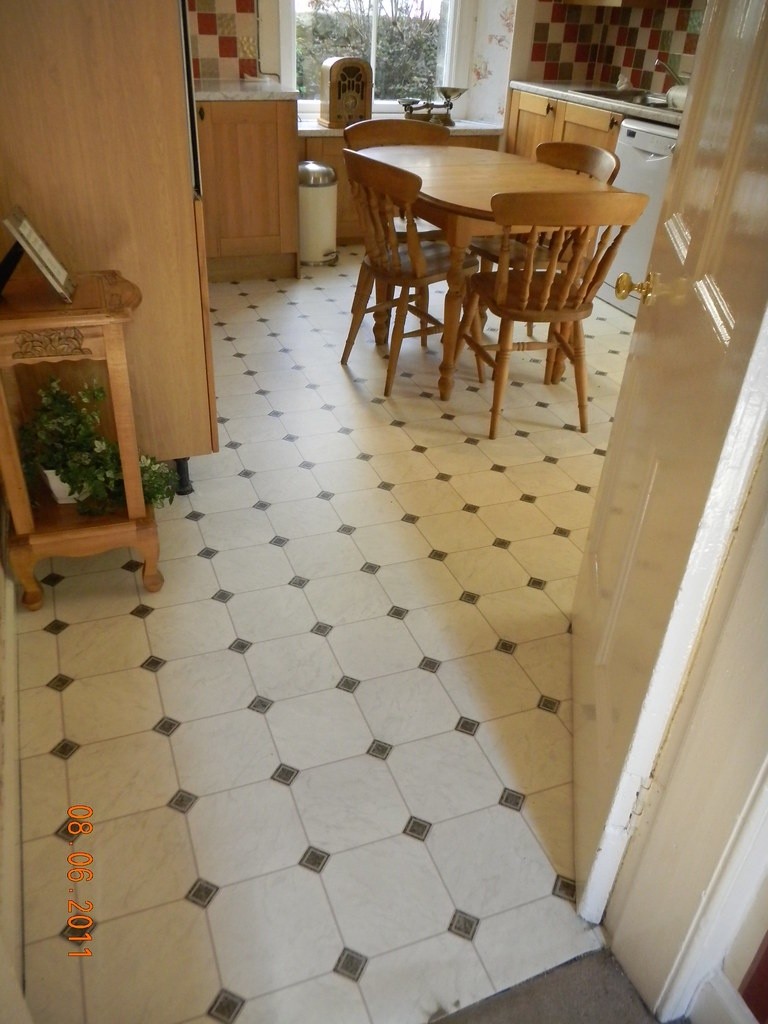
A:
[639,101,684,115]
[568,89,667,107]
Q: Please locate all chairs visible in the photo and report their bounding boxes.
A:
[342,119,620,439]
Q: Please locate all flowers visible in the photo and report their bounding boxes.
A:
[16,375,180,516]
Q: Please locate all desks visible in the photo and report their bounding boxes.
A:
[357,144,628,401]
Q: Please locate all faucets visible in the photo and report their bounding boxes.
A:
[653,58,684,85]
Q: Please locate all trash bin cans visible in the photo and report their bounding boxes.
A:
[298,159,340,268]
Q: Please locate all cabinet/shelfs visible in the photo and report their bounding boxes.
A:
[0,1,219,496]
[0,269,164,610]
[196,101,300,283]
[507,90,625,263]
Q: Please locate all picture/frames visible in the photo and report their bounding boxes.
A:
[2,204,76,304]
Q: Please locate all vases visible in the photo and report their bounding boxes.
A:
[37,464,79,504]
